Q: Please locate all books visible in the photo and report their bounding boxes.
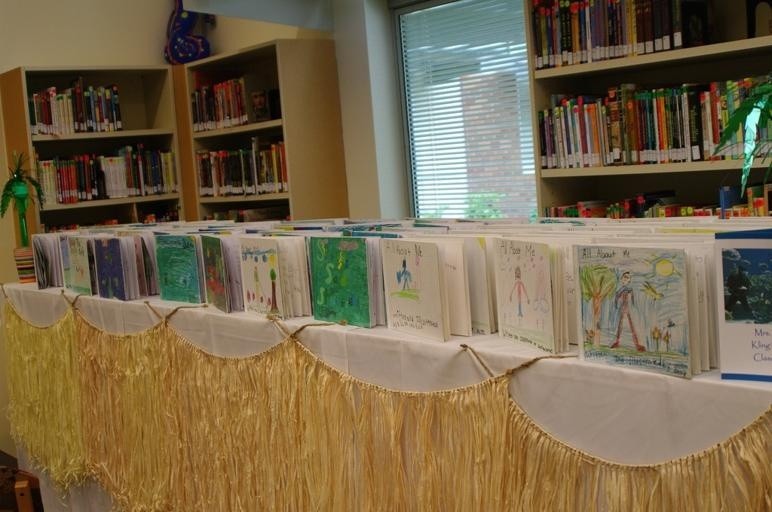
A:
[137,210,178,223]
[378,211,772,382]
[190,75,283,133]
[204,206,291,219]
[537,72,772,171]
[31,219,398,327]
[529,0,709,70]
[194,137,288,196]
[37,144,180,206]
[26,77,123,137]
[41,219,119,233]
[547,181,772,219]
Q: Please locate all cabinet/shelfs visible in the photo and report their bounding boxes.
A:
[524,0,772,217]
[0,35,349,247]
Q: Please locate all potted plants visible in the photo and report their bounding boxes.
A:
[0,151,44,248]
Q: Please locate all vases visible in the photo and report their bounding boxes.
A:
[15,248,34,281]
